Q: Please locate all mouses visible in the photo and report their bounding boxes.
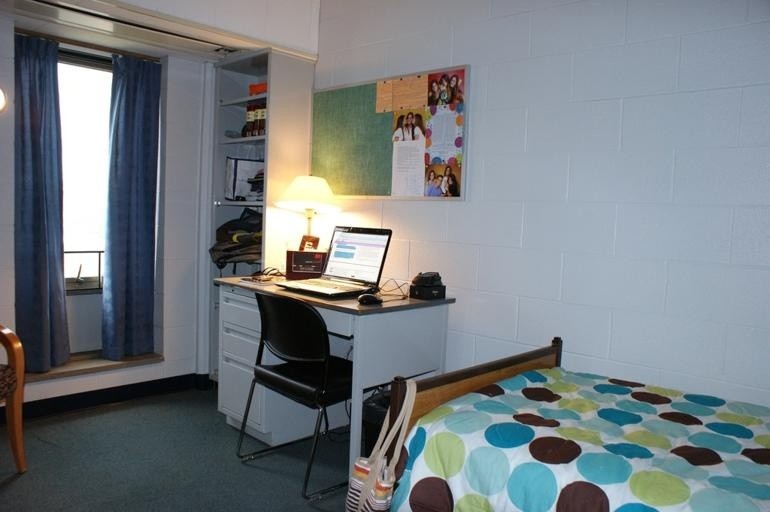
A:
[357,294,383,306]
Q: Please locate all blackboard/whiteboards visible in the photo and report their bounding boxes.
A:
[309,63,472,203]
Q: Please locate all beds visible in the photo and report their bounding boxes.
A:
[379,333,769,512]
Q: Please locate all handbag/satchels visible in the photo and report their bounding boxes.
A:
[344,457,396,512]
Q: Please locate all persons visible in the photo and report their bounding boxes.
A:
[429,75,464,104]
[426,166,458,197]
[392,113,426,141]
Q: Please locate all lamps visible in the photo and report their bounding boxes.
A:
[275,175,341,235]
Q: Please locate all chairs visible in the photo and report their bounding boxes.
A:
[0,323,28,474]
[235,292,352,501]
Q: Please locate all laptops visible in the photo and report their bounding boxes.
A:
[275,225,392,299]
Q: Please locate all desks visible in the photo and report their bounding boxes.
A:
[213,272,457,493]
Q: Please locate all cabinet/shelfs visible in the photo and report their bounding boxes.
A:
[208,46,316,386]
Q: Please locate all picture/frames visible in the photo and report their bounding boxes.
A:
[298,235,321,252]
